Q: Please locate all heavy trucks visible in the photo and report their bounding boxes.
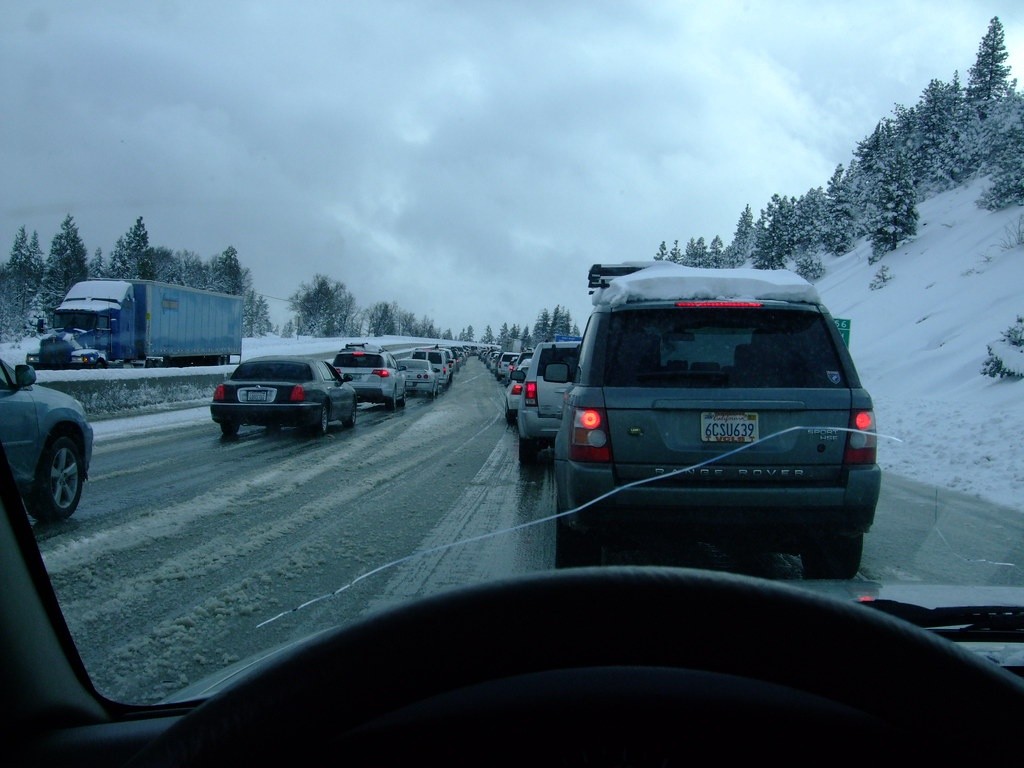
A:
[26,279,244,369]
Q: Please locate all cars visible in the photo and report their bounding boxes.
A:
[396,360,438,397]
[408,344,471,389]
[471,339,583,466]
[0,358,93,523]
[210,355,358,439]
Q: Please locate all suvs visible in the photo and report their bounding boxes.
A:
[332,342,407,413]
[543,264,882,584]
